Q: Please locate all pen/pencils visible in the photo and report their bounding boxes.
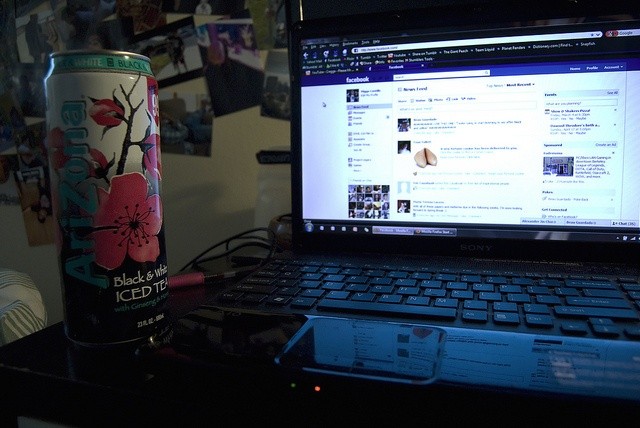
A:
[168,270,237,288]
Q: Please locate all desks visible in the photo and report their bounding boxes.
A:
[1,256,640,428]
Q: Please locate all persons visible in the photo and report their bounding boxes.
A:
[25,13,53,63]
[168,30,188,74]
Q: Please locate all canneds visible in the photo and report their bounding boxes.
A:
[43,48,173,347]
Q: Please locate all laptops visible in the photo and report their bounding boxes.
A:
[129,1,640,415]
